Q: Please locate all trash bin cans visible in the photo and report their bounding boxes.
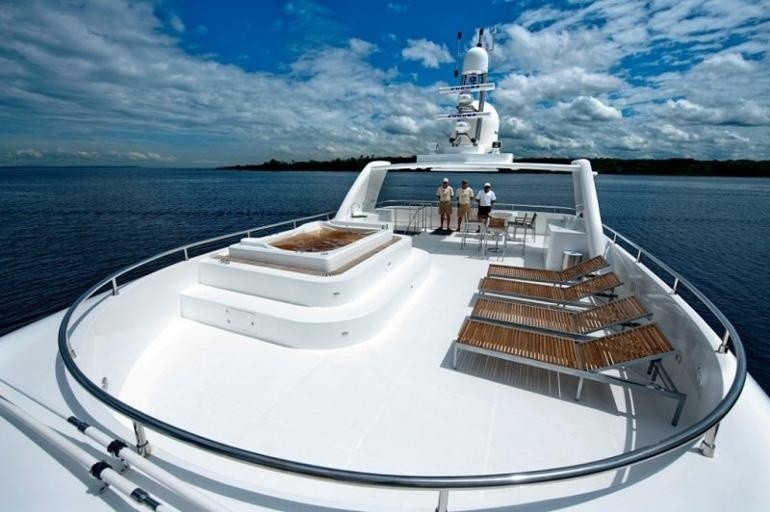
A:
[561,251,583,281]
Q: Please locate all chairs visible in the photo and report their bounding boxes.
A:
[487,254,610,288]
[471,295,654,341]
[453,316,688,427]
[478,271,623,312]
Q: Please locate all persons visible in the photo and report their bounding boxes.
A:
[475,182,497,234]
[436,177,455,234]
[455,178,475,231]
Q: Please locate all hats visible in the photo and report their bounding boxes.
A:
[443,177,449,183]
[484,182,491,187]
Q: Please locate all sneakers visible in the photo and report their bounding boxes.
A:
[438,227,461,232]
